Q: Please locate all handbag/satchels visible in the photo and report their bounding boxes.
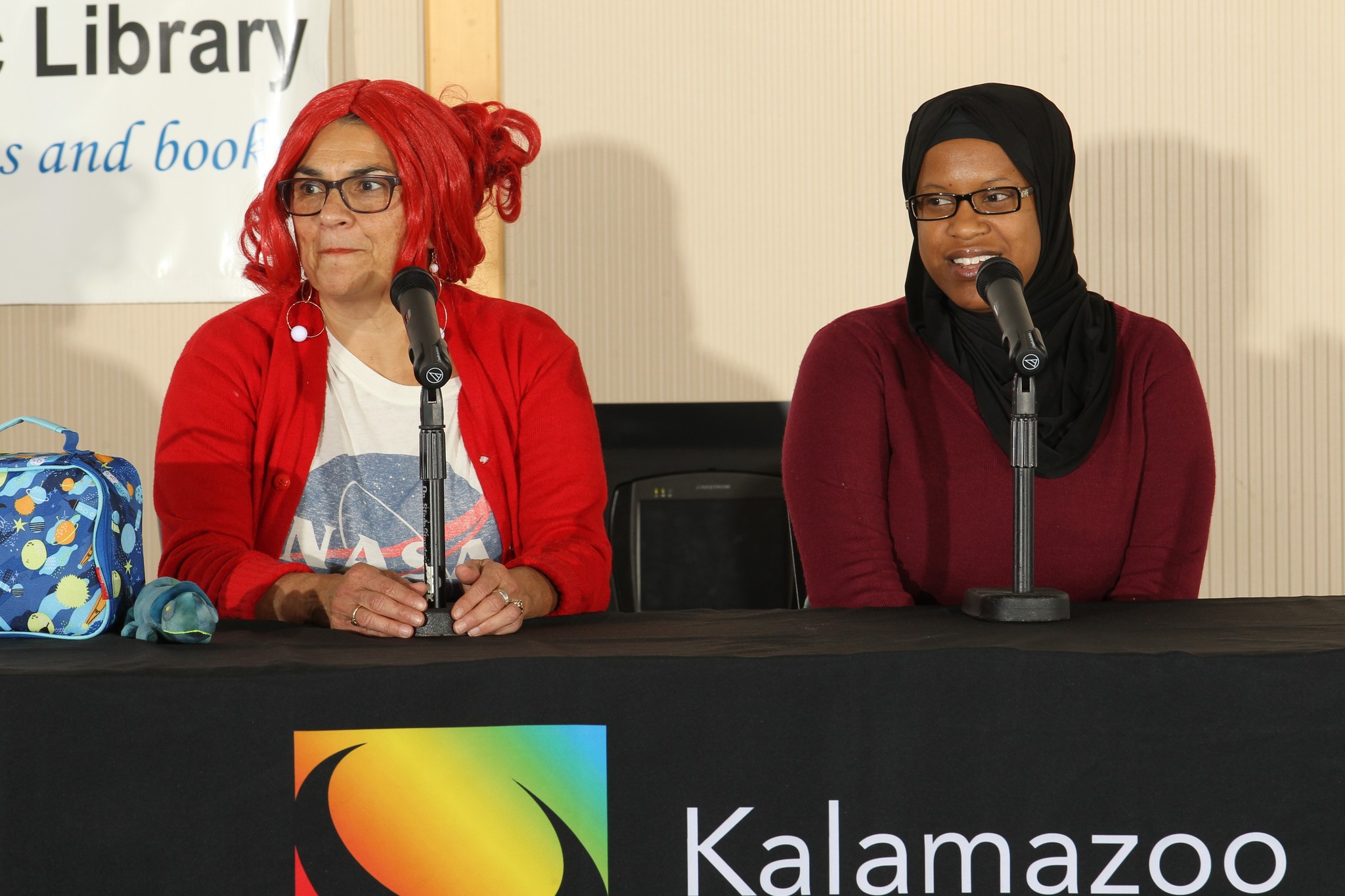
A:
[0,417,146,641]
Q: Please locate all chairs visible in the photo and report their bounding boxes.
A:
[608,467,806,611]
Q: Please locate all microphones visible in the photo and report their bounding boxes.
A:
[389,265,452,390]
[976,257,1049,378]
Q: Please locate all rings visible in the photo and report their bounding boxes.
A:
[350,605,361,627]
[508,598,525,615]
[494,589,509,606]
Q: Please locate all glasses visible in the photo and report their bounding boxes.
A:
[276,175,402,216]
[905,183,1033,221]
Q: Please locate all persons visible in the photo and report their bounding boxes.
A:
[151,77,612,641]
[782,81,1218,610]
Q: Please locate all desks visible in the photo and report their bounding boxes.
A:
[0,594,1344,896]
